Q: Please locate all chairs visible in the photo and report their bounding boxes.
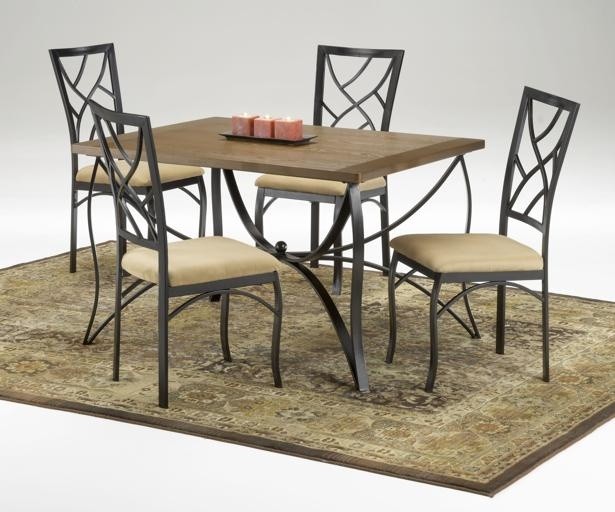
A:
[87,98,283,409]
[47,42,207,274]
[385,84,581,393]
[254,44,404,295]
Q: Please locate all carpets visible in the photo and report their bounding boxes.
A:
[0,238,615,498]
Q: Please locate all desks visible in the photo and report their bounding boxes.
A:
[71,116,485,392]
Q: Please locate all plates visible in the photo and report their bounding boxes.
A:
[220,130,317,144]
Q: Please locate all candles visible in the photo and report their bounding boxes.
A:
[231,115,305,141]
